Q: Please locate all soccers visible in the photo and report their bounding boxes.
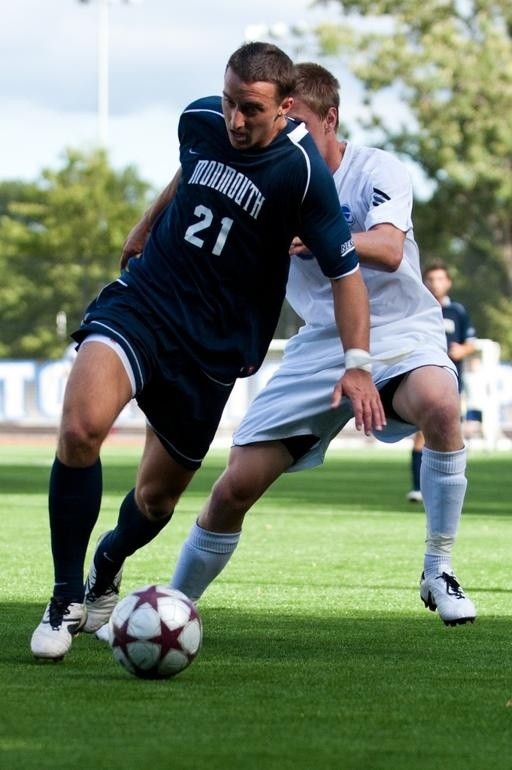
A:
[107,585,204,679]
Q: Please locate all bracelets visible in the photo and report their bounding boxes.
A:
[342,348,373,375]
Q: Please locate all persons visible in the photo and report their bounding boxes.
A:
[25,37,391,661]
[403,258,485,504]
[457,354,489,448]
[170,60,484,636]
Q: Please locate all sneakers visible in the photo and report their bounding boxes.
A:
[421,571,475,626]
[408,491,422,502]
[31,530,123,661]
